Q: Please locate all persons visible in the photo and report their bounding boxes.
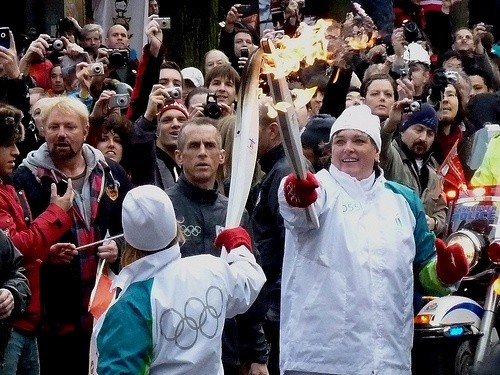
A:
[149,117,272,375]
[274,105,472,375]
[9,94,124,375]
[96,183,269,375]
[0,227,32,375]
[0,100,77,375]
[0,0,500,375]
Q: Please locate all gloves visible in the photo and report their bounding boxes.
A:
[214,225,252,253]
[284,171,319,208]
[435,238,469,286]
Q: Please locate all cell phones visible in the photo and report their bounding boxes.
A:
[240,48,248,65]
[0,27,9,49]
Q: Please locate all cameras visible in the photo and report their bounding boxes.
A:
[402,100,422,113]
[108,93,129,108]
[44,37,64,51]
[82,62,104,76]
[103,48,128,68]
[402,21,424,44]
[152,16,171,29]
[483,25,493,32]
[161,86,183,99]
[236,4,252,12]
[199,91,222,119]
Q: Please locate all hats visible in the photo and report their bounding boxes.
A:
[158,101,190,122]
[403,42,431,67]
[300,113,336,149]
[401,104,439,138]
[180,67,205,88]
[121,185,177,251]
[329,104,381,155]
[0,102,25,144]
